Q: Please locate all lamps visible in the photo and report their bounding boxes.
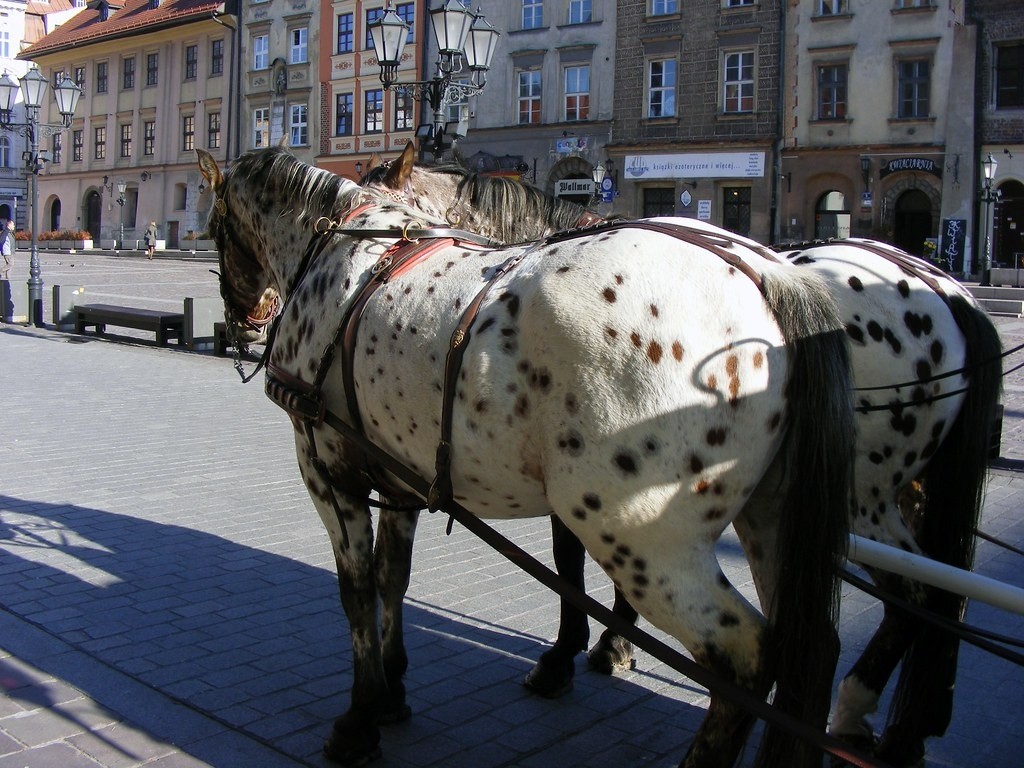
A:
[604,157,618,192]
[354,162,368,179]
[861,158,871,183]
[103,174,113,197]
[140,170,151,181]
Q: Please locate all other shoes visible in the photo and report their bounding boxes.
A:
[150,257,152,260]
[0,316,12,324]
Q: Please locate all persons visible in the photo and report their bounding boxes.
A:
[143,221,157,260]
[0,220,15,280]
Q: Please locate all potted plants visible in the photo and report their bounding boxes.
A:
[181,228,217,252]
[12,230,94,249]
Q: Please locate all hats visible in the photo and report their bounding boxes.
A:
[151,221,156,226]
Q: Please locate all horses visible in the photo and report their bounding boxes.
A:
[192,132,1005,768]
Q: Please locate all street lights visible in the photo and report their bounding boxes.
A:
[978,152,1001,286]
[0,62,84,328]
[116,179,128,251]
[365,0,501,167]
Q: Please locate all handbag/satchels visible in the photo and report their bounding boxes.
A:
[144,232,150,240]
